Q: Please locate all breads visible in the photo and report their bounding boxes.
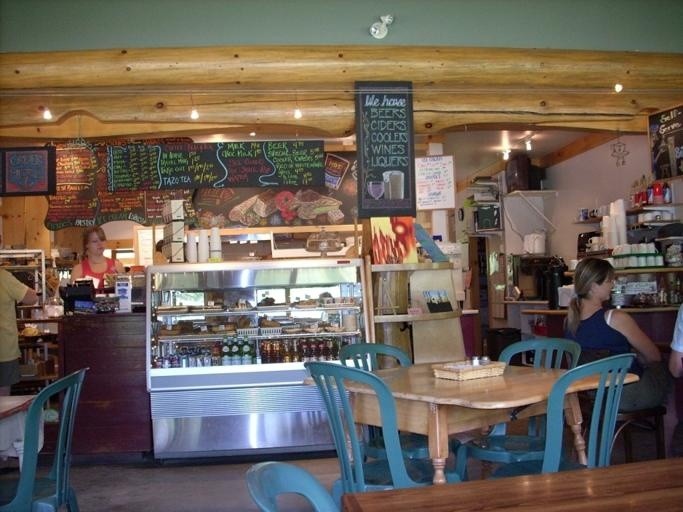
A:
[155,300,345,336]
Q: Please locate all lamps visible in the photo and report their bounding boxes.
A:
[190,94,199,120]
[43,96,52,120]
[294,94,302,119]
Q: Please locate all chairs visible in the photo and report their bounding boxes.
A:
[482,328,533,365]
[244,462,340,512]
[338,343,468,483]
[574,348,667,466]
[493,353,638,480]
[0,368,87,512]
[304,361,461,512]
[465,336,582,480]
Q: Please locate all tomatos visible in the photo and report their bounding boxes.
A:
[276,191,297,222]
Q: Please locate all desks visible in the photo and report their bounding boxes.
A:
[0,395,45,473]
[342,362,641,485]
[342,458,683,512]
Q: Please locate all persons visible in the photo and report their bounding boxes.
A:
[69,227,126,295]
[563,258,672,413]
[666,302,683,458]
[0,268,37,396]
[491,253,505,285]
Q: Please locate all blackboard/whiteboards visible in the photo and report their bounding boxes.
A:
[1,146,54,195]
[415,153,456,211]
[354,83,415,218]
[107,140,327,189]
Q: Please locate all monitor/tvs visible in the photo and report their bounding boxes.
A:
[58,279,95,316]
[504,154,547,193]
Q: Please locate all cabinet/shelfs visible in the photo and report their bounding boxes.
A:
[365,261,467,369]
[520,205,683,350]
[137,258,369,461]
[0,248,59,409]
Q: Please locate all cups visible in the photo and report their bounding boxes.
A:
[577,231,602,252]
[571,203,601,221]
[613,243,665,267]
[602,198,630,249]
[627,209,676,225]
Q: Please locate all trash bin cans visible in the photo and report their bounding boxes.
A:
[488,328,522,366]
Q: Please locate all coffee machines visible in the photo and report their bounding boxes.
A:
[505,254,563,303]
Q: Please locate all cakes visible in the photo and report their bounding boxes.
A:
[298,188,345,224]
[193,187,276,227]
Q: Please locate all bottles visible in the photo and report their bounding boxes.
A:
[466,354,490,366]
[151,334,257,368]
[657,273,683,307]
[185,227,224,263]
[630,174,672,205]
[259,335,363,364]
